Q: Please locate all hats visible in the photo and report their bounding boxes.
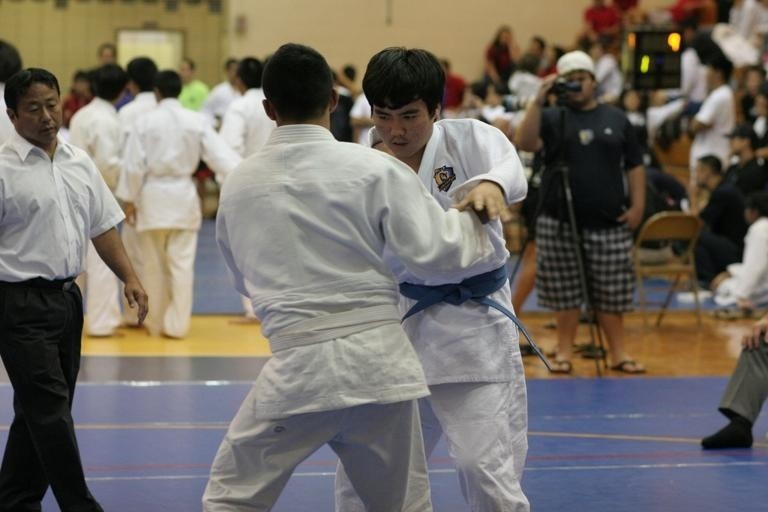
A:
[556,51,598,81]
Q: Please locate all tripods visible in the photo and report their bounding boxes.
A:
[510,107,607,377]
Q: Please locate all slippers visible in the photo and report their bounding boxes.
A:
[549,354,573,374]
[603,359,647,374]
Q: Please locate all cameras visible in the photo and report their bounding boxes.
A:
[546,76,581,113]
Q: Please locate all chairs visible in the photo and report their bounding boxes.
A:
[628,211,707,336]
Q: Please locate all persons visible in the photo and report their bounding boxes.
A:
[1,66,149,512]
[201,41,537,512]
[0,0,768,376]
[699,312,766,454]
